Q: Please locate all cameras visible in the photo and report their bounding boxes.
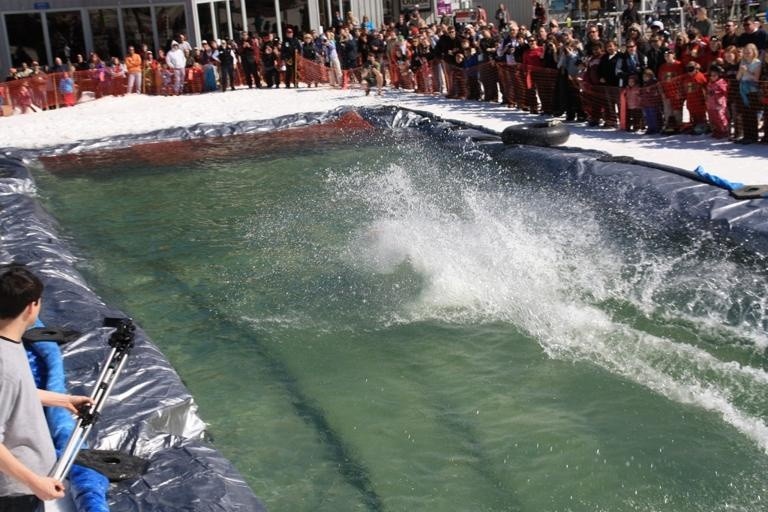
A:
[366,60,372,65]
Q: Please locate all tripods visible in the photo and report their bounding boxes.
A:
[49,317,136,492]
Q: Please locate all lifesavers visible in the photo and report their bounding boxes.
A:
[501,121,569,145]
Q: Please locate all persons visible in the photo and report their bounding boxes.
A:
[1,1,768,147]
[1,262,96,512]
